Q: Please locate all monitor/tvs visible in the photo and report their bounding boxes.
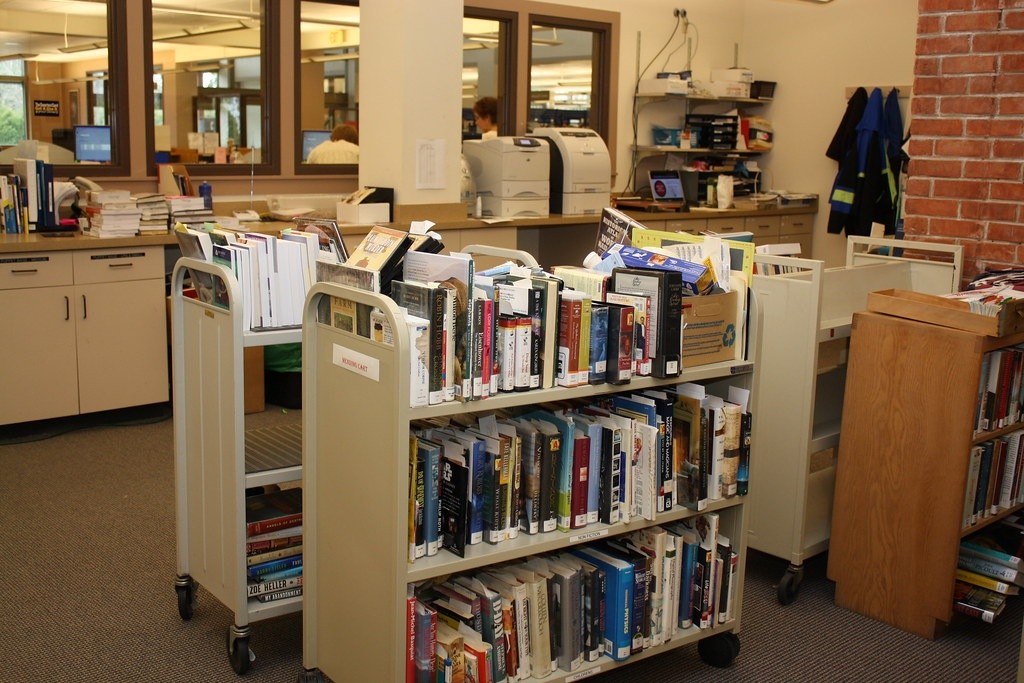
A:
[301,130,334,162]
[52,129,75,151]
[73,125,111,163]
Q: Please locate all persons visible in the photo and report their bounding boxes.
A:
[306,123,359,164]
[473,97,498,140]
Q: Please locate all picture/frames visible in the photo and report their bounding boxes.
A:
[68,88,81,127]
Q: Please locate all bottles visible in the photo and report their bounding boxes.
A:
[199,180,213,208]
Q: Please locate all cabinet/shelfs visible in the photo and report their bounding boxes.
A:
[745,236,966,606]
[638,220,666,231]
[0,244,171,426]
[629,92,770,154]
[433,229,461,257]
[744,216,779,246]
[461,227,518,273]
[665,219,707,238]
[686,114,739,149]
[825,311,1024,643]
[708,217,745,235]
[681,169,762,203]
[296,282,765,683]
[779,214,815,271]
[171,257,304,674]
[340,234,365,257]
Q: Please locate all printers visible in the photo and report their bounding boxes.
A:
[527,127,611,215]
[463,137,550,218]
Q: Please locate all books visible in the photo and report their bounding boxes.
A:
[352,206,801,407]
[2,155,57,235]
[244,486,304,602]
[216,218,350,328]
[952,346,1024,623]
[408,408,753,683]
[80,190,212,304]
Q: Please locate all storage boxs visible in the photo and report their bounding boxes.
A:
[637,66,754,101]
[336,202,391,224]
[682,291,737,370]
[365,186,394,223]
[765,193,819,215]
